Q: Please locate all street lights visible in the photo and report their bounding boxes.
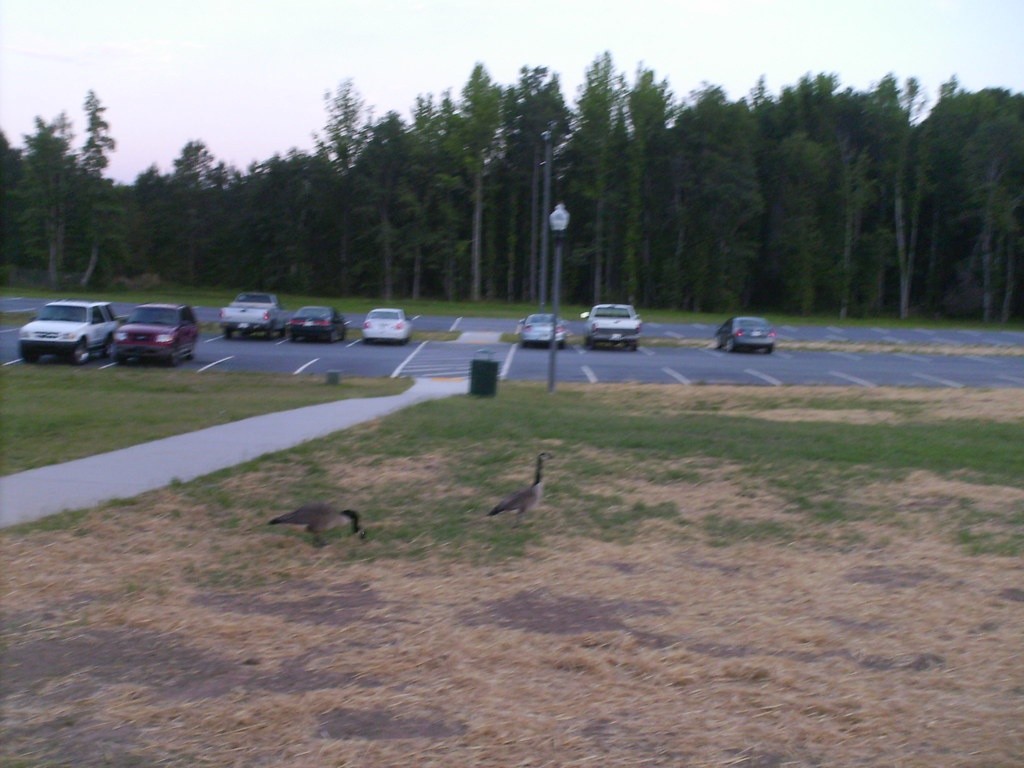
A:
[546,201,571,395]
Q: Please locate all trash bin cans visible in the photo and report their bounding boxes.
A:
[469,349,500,397]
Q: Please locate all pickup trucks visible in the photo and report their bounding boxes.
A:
[16,300,129,367]
[217,292,288,342]
[580,303,642,352]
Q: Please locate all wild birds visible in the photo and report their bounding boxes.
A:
[265,500,362,544]
[487,452,556,529]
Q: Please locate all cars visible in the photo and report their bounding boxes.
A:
[361,307,420,346]
[713,315,776,355]
[518,313,569,350]
[110,303,200,366]
[287,305,352,344]
